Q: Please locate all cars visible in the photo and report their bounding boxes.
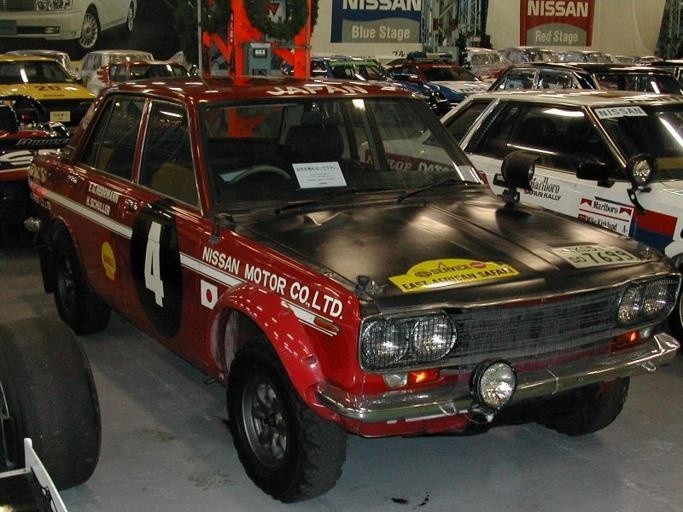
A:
[76,50,154,97]
[1,99,78,234]
[0,1,142,56]
[278,46,682,122]
[27,78,681,503]
[5,49,79,82]
[0,55,98,135]
[106,61,189,84]
[359,88,682,332]
